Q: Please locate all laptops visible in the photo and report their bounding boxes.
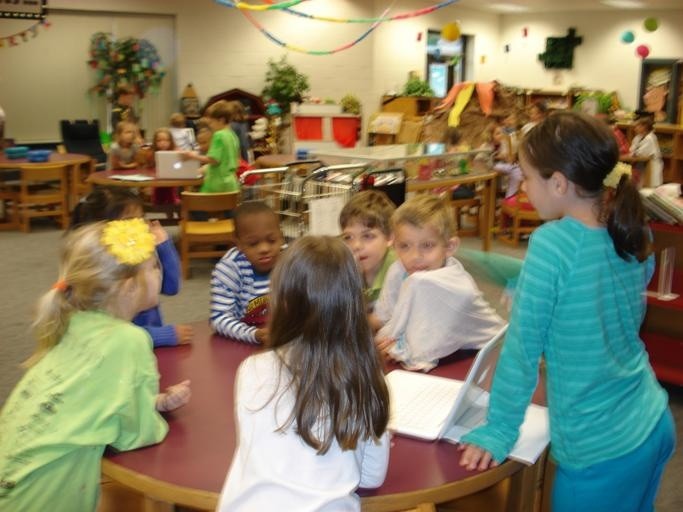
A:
[154,148,202,180]
[386,324,508,444]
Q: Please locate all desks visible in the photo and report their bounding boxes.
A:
[92,315,552,512]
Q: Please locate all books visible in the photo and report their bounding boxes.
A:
[442,389,557,467]
[637,176,682,229]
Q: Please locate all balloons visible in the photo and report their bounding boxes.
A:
[637,45,649,58]
[622,31,634,44]
[644,17,657,32]
[442,22,459,41]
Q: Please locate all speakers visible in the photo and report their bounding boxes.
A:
[544,37,573,68]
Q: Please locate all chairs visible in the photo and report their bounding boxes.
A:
[61,118,106,164]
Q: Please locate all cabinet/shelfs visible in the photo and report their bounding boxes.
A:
[611,121,682,186]
[190,87,446,144]
[527,88,585,110]
[623,222,683,394]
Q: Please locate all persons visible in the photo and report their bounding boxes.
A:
[217,236,391,510]
[211,200,288,344]
[367,197,512,373]
[105,100,255,268]
[520,103,548,138]
[454,108,678,511]
[473,124,507,162]
[601,117,631,157]
[73,187,195,347]
[497,110,524,133]
[0,217,193,511]
[338,189,397,311]
[626,110,666,188]
